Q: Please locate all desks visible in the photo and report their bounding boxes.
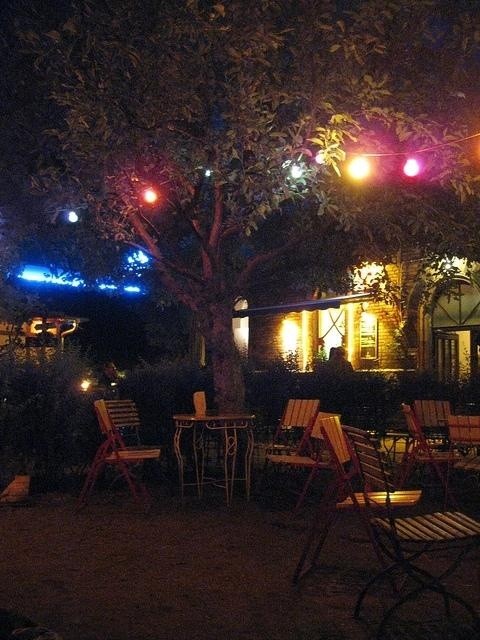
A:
[173,413,256,509]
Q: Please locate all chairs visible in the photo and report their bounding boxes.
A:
[320,415,478,638]
[263,396,480,581]
[75,399,161,512]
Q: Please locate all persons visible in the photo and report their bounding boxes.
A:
[96,358,118,387]
[312,346,362,473]
